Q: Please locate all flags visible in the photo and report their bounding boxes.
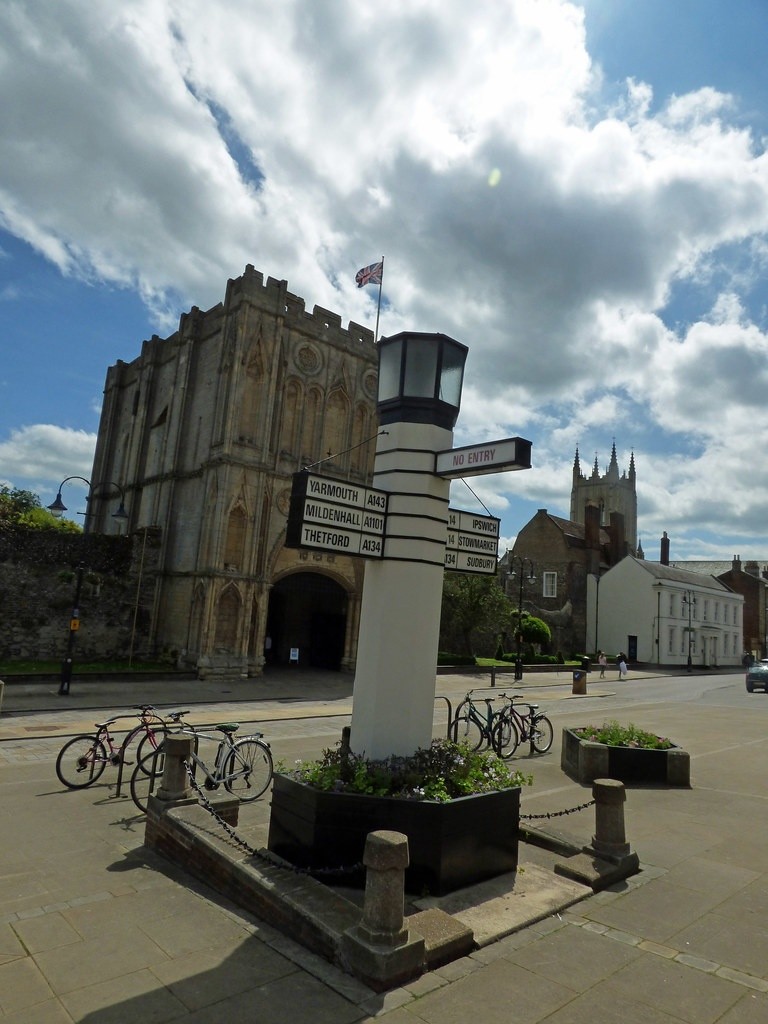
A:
[356,262,382,288]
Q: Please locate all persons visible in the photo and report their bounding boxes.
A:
[616,651,627,678]
[598,652,608,679]
[742,649,754,669]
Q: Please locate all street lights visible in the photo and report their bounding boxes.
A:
[506,555,537,679]
[681,589,697,672]
[47,477,129,696]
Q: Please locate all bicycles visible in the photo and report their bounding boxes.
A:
[448,690,512,752]
[491,693,553,759]
[130,711,273,813]
[55,704,173,789]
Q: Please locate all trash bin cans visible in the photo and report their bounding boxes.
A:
[582,656,592,673]
[0,680,5,704]
[572,669,587,695]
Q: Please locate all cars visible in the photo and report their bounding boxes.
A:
[746,667,768,693]
